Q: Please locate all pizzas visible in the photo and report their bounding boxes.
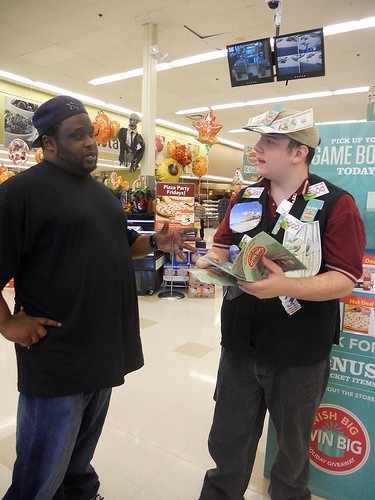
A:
[342,311,370,332]
[155,200,195,218]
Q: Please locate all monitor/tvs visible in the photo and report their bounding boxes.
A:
[274,27,325,82]
[226,37,275,88]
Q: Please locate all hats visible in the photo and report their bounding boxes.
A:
[241,111,320,149]
[32,95,87,147]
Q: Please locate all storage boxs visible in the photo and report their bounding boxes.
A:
[163,248,216,299]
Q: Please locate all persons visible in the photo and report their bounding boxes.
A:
[198,105,367,500]
[0,95,197,500]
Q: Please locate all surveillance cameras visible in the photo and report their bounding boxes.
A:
[266,0,280,9]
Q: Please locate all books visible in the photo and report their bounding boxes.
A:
[199,231,307,282]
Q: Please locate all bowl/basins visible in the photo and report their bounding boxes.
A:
[4,101,35,143]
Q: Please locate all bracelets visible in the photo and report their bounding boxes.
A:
[150,233,161,253]
